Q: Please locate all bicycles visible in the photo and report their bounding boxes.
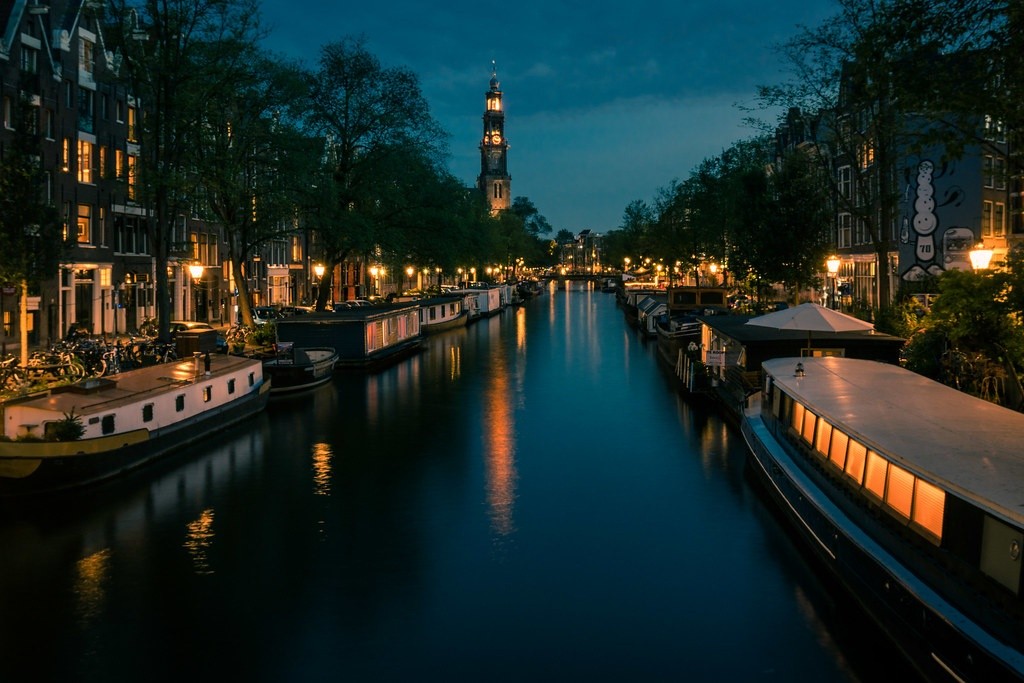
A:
[0,332,178,395]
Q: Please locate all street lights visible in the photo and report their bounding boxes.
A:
[189,260,206,322]
[826,255,841,309]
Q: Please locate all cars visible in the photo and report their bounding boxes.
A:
[279,307,314,317]
[153,322,229,354]
[238,307,282,328]
[332,300,372,312]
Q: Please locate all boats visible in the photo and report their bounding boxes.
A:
[405,282,540,333]
[263,343,339,394]
[269,303,425,364]
[696,304,906,398]
[0,351,273,493]
[739,357,1024,683]
[653,314,702,338]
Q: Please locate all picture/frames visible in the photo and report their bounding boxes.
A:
[77,222,86,236]
